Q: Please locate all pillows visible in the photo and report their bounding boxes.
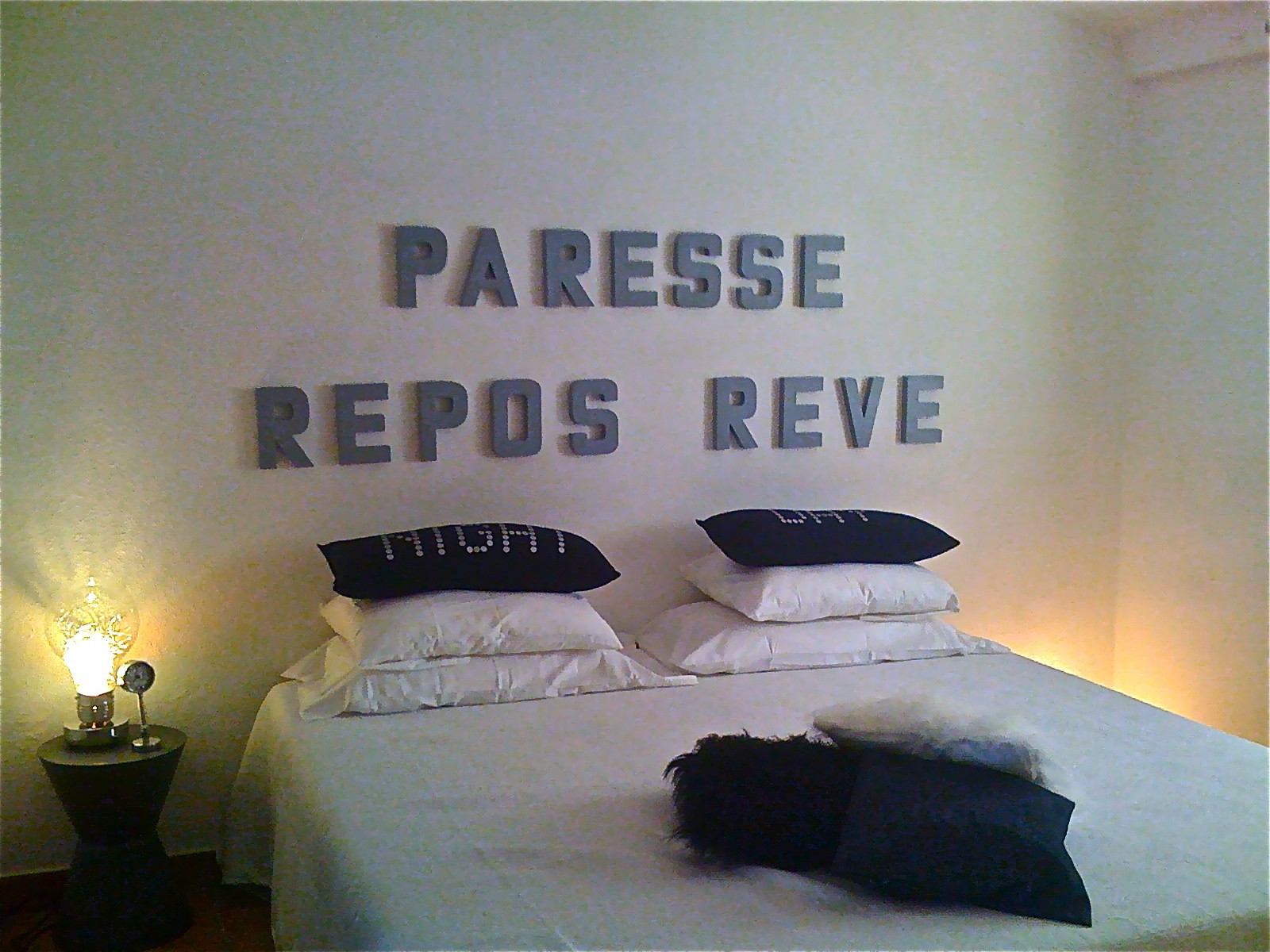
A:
[278,510,976,721]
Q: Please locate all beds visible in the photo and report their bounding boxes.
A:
[216,638,1270,952]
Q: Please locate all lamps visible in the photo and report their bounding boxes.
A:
[42,577,139,747]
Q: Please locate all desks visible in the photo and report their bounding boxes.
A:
[36,724,192,952]
